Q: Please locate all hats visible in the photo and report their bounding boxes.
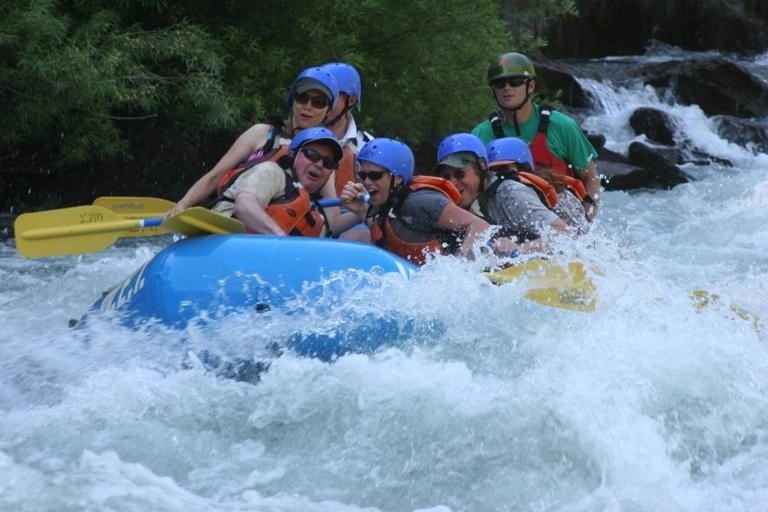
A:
[433,152,485,178]
[300,136,342,162]
[487,160,517,169]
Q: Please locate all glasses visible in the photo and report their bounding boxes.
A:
[301,149,339,170]
[357,170,389,181]
[494,79,530,89]
[442,168,471,179]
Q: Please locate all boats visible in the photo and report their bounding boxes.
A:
[68,229,762,396]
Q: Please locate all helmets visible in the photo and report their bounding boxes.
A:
[356,137,415,189]
[288,62,361,112]
[288,126,338,159]
[488,52,537,86]
[437,132,489,172]
[485,137,535,168]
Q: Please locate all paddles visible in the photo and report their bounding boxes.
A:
[15,198,341,256]
[483,259,597,312]
[93,197,244,238]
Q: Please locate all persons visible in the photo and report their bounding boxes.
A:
[471,52,600,222]
[486,137,591,236]
[356,136,489,269]
[340,133,567,258]
[210,127,344,238]
[320,62,378,242]
[163,66,362,235]
